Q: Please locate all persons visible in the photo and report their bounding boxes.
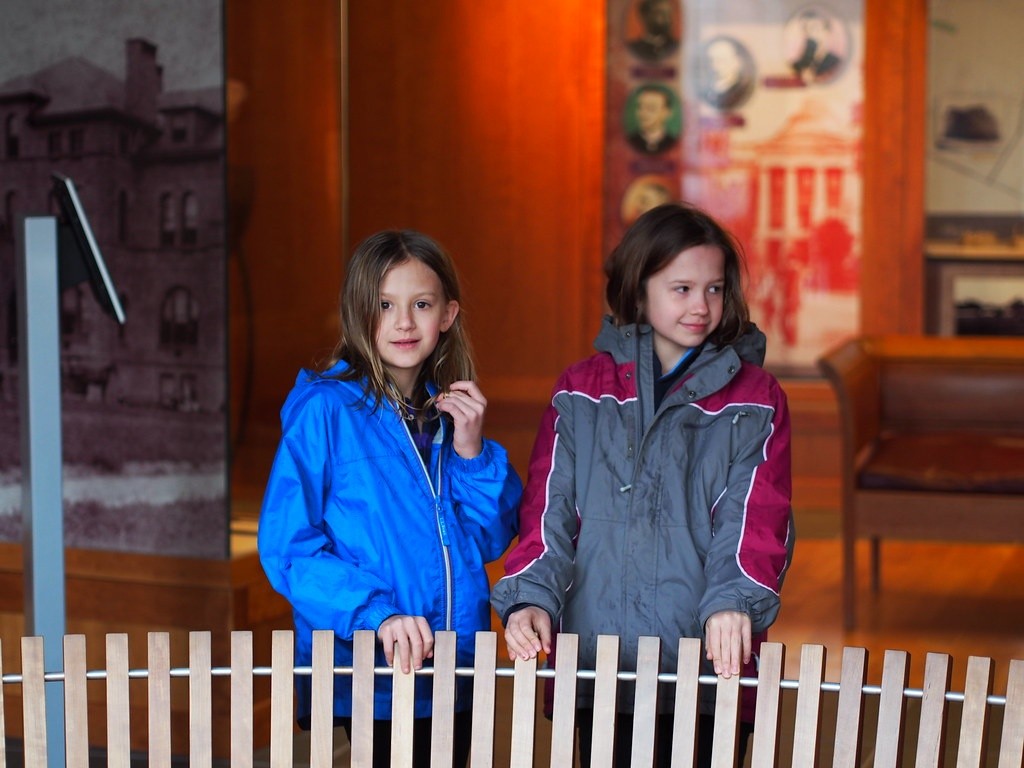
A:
[793,8,846,84]
[488,204,801,768]
[632,182,668,216]
[627,88,678,156]
[700,36,751,104]
[255,229,525,767]
[618,0,677,63]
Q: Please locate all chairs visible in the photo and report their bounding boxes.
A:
[818,332,1024,637]
